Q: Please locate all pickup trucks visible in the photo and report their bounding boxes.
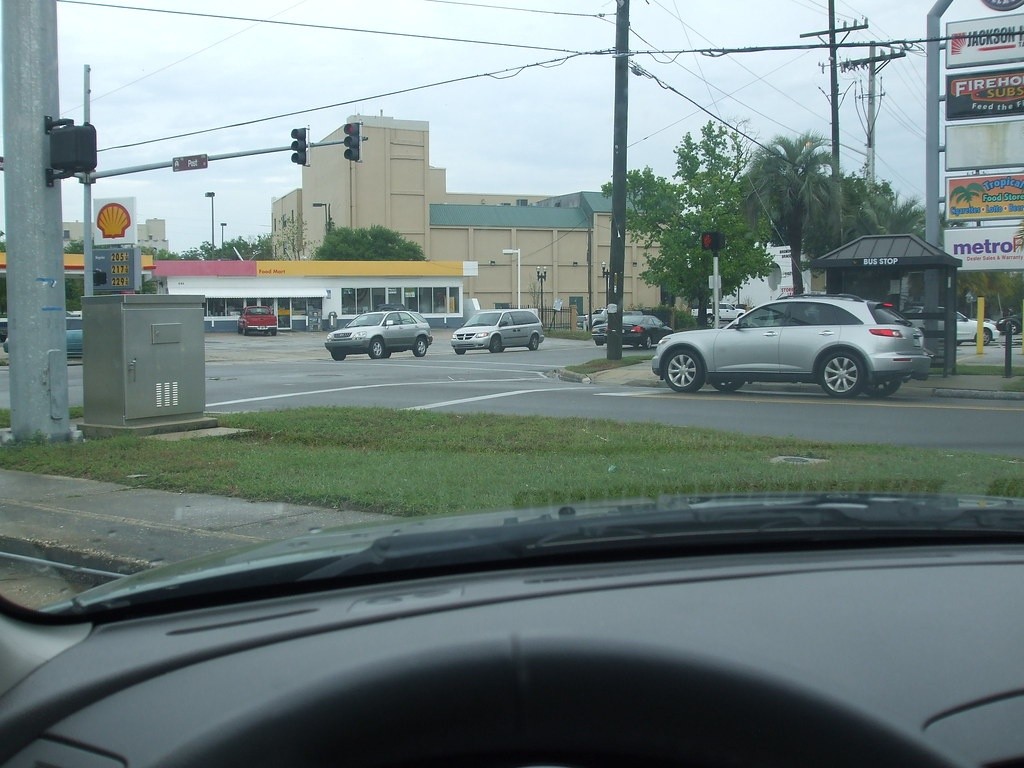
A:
[576,308,607,328]
[237,306,277,336]
[691,303,746,324]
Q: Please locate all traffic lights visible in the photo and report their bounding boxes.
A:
[343,123,358,161]
[290,127,306,164]
[702,232,725,252]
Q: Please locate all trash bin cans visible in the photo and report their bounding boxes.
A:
[328,311,338,331]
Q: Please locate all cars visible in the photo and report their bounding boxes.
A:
[374,303,412,324]
[970,318,997,329]
[901,306,1000,345]
[0,322,8,340]
[997,313,1023,334]
[592,311,643,346]
[605,315,673,350]
[3,317,83,358]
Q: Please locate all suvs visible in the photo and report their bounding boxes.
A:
[650,292,931,397]
[325,311,433,361]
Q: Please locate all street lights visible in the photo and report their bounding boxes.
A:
[537,266,546,324]
[966,291,978,318]
[205,192,215,259]
[313,203,328,235]
[602,261,608,307]
[221,223,227,258]
[502,249,520,309]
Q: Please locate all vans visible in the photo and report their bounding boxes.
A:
[451,310,544,354]
